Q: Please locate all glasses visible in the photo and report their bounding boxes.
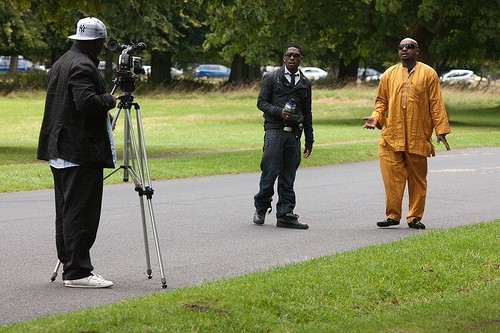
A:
[397,44,417,50]
[284,52,302,57]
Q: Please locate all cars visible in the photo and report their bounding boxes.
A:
[259,65,281,77]
[139,65,184,80]
[192,64,231,77]
[95,61,119,73]
[440,70,477,87]
[301,66,329,81]
[0,56,34,73]
[353,67,381,83]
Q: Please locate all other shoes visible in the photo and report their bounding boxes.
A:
[408,219,425,229]
[376,217,400,227]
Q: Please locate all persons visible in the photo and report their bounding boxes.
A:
[362,37,451,230]
[253,43,314,230]
[36,17,116,288]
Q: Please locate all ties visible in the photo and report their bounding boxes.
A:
[290,74,295,87]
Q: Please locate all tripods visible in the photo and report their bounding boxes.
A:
[51,102,168,289]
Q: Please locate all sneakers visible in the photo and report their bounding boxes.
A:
[62,272,113,288]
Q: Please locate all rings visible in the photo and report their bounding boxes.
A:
[286,115,289,118]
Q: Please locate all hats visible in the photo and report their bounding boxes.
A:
[68,16,107,40]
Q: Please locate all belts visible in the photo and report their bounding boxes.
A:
[282,126,299,132]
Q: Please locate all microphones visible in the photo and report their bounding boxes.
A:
[281,99,296,127]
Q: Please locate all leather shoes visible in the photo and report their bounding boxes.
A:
[277,213,309,229]
[253,207,267,225]
